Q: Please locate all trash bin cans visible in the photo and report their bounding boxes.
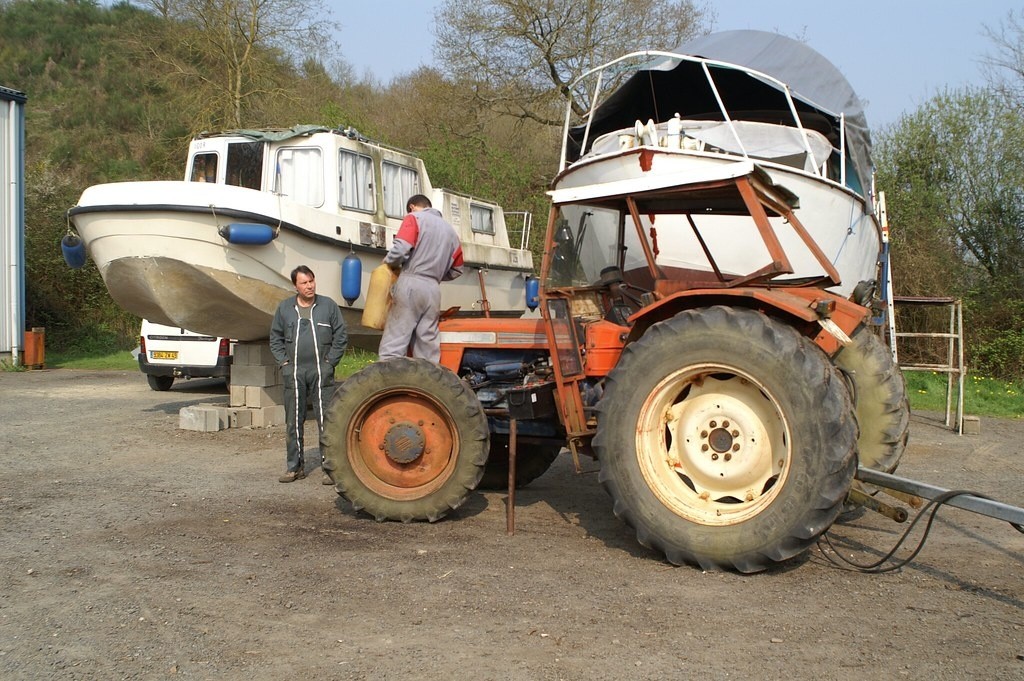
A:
[23,327,47,370]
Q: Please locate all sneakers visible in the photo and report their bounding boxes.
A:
[278,468,305,483]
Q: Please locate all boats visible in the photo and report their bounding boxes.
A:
[64,115,557,346]
[548,28,900,357]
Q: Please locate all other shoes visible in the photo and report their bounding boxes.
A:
[321,472,332,485]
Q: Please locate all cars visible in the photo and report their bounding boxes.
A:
[136,317,239,392]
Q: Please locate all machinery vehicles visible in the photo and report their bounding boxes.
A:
[319,165,915,579]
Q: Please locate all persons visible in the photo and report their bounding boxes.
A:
[271,266,349,485]
[378,194,463,363]
